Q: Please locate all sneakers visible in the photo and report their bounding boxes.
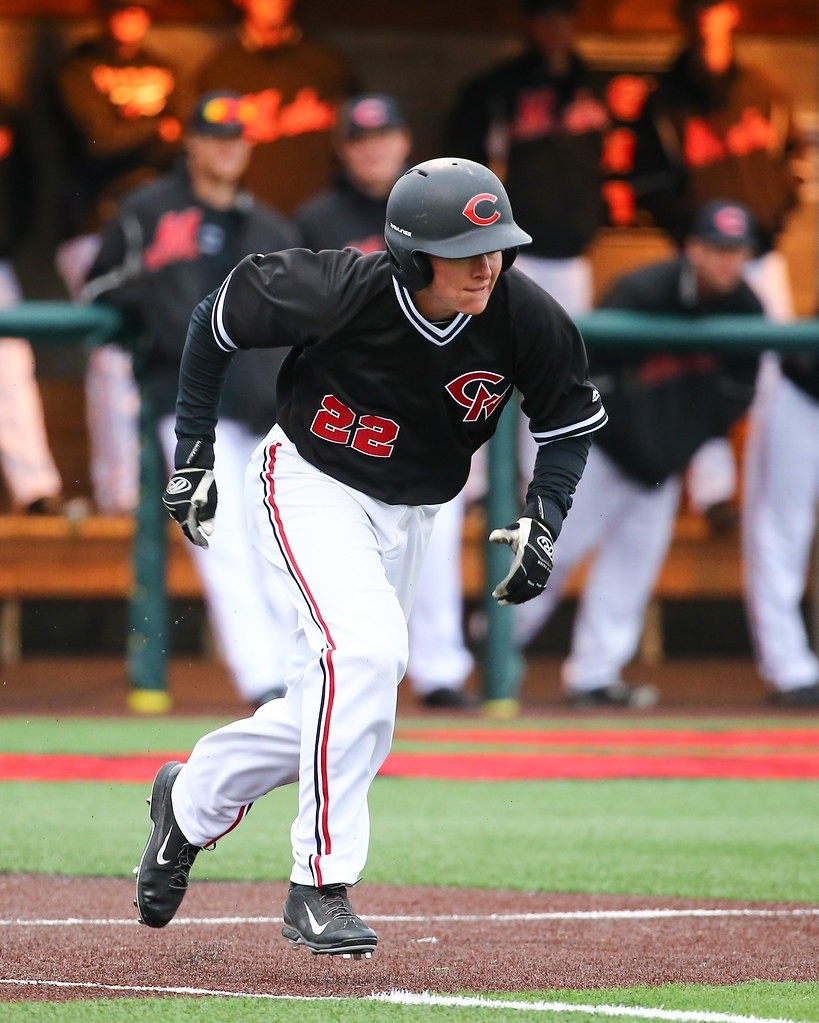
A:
[132,760,217,929]
[281,876,379,959]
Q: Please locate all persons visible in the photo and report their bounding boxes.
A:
[133,156,608,960]
[0,1,819,717]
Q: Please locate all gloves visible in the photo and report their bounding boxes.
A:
[160,437,218,550]
[488,493,566,607]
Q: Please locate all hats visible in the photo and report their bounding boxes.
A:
[330,93,412,145]
[689,198,764,251]
[187,90,260,141]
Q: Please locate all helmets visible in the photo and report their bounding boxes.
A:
[383,157,533,292]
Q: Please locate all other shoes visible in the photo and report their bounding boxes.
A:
[421,689,480,709]
[567,680,644,711]
[766,682,819,709]
